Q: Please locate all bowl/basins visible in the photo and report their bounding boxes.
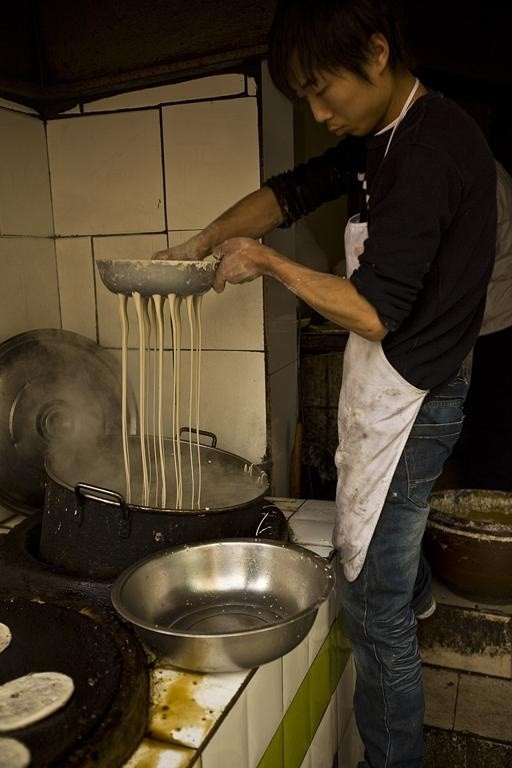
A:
[112,537,337,675]
[94,258,221,297]
[426,487,512,606]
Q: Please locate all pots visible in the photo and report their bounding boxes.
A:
[40,426,272,548]
[0,583,152,768]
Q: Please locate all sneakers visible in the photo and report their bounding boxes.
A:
[417,598,438,619]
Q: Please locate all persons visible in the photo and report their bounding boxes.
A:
[151,1,499,768]
